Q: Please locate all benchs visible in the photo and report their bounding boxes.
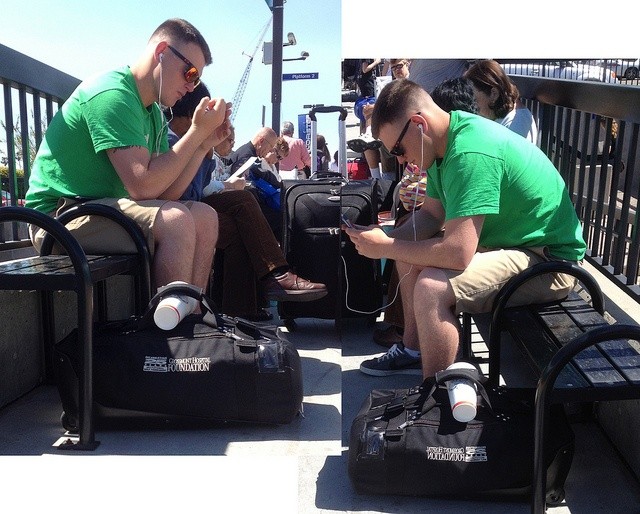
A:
[1,199,157,449]
[463,261,639,513]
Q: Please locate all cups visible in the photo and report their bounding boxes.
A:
[444,361,478,422]
[154,281,198,330]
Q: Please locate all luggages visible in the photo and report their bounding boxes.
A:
[277,103,382,332]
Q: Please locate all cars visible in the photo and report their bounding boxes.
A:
[0,189,26,207]
[584,59,640,80]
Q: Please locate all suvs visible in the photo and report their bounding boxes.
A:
[493,59,617,84]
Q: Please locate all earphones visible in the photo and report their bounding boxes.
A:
[158,53,164,63]
[418,123,424,135]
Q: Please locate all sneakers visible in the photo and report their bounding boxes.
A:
[361,342,420,376]
[373,326,403,346]
[239,309,273,321]
[263,272,328,301]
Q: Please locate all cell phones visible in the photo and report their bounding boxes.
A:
[340,212,354,229]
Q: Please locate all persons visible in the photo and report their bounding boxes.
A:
[22,16,234,314]
[429,78,479,114]
[264,137,290,181]
[231,126,283,191]
[317,134,329,171]
[347,59,411,179]
[341,78,589,384]
[276,122,311,180]
[162,78,329,322]
[354,58,383,136]
[372,78,481,348]
[463,59,539,149]
[201,123,246,199]
[330,150,338,172]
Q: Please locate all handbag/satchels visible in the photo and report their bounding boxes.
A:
[54,283,304,431]
[347,158,370,181]
[254,178,280,210]
[349,370,577,507]
[399,163,427,211]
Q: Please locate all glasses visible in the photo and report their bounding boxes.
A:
[168,44,202,87]
[390,112,424,157]
[390,63,407,70]
[274,148,283,160]
